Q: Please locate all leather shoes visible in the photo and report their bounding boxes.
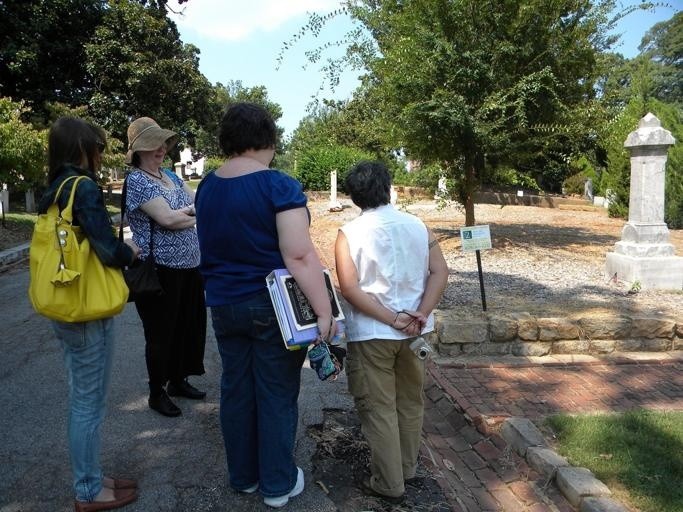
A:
[96,476,139,489]
[74,487,137,512]
[167,382,208,401]
[148,389,182,418]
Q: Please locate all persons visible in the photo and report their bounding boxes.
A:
[194,101,336,507]
[30,118,138,511]
[124,116,207,416]
[335,164,449,499]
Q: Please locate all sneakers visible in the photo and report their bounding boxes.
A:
[356,472,405,504]
[240,483,259,495]
[405,475,417,484]
[265,465,305,509]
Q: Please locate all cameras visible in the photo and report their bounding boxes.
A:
[408,338,435,363]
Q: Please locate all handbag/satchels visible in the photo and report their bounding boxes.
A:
[116,172,161,302]
[27,174,130,323]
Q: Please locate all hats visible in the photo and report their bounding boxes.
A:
[124,116,180,164]
